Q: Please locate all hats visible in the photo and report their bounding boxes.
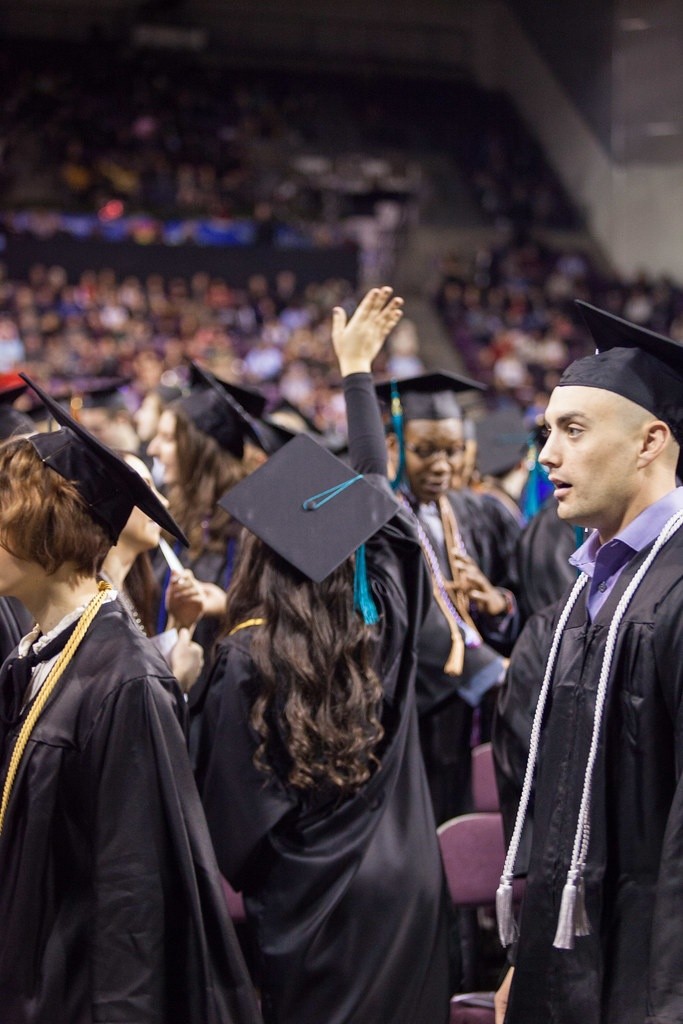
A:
[194,358,282,456]
[554,299,683,435]
[15,371,187,555]
[218,434,399,625]
[373,369,486,495]
[474,400,533,477]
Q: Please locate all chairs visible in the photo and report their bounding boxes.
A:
[451,991,499,1024]
[436,813,509,903]
[219,871,247,918]
[474,739,503,812]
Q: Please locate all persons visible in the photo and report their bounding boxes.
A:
[0,372,264,1023]
[490,299,683,1024]
[0,46,683,1002]
[185,284,464,1024]
